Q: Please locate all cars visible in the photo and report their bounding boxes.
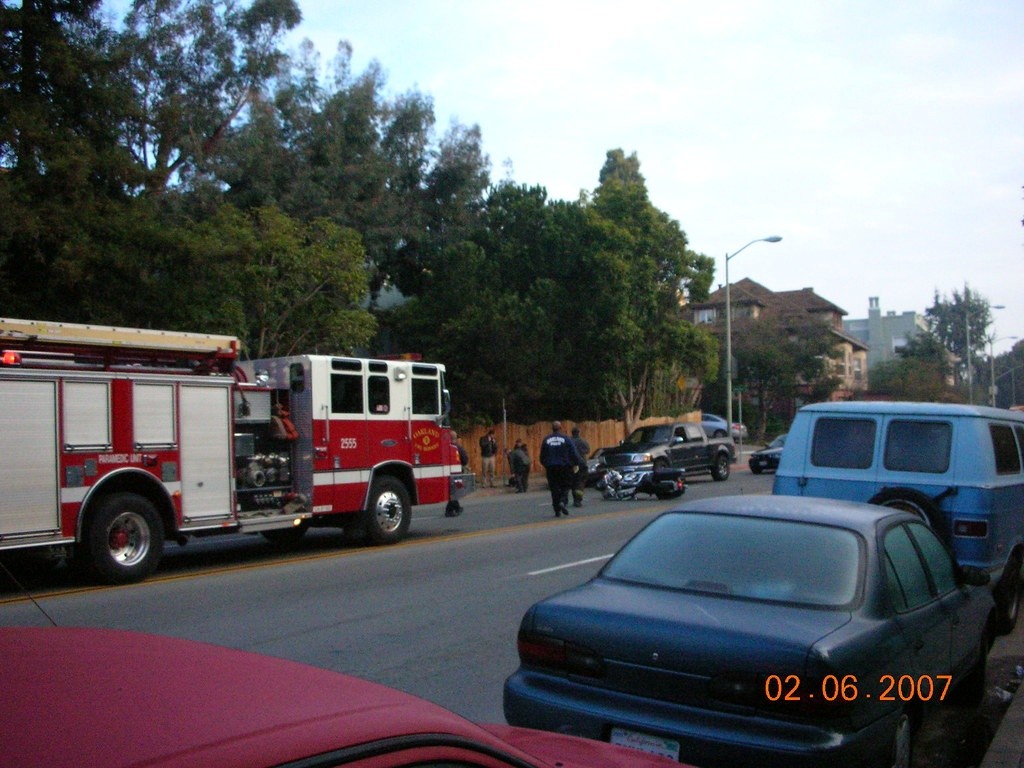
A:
[502,492,998,768]
[574,446,609,487]
[748,433,789,475]
[701,412,749,441]
[1,624,690,768]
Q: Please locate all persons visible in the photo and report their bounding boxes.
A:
[445,421,529,517]
[538,421,583,518]
[567,427,590,508]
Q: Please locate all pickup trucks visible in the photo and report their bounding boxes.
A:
[597,421,738,485]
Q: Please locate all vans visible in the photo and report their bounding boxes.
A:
[772,400,1024,638]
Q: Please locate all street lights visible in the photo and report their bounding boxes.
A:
[966,304,1005,404]
[990,336,1018,407]
[726,234,783,439]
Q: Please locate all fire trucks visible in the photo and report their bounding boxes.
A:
[1,319,462,586]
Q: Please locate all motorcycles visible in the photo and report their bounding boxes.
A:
[597,464,687,501]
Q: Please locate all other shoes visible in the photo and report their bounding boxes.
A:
[482,484,486,488]
[555,509,561,517]
[445,504,463,517]
[560,502,569,515]
[490,485,497,488]
